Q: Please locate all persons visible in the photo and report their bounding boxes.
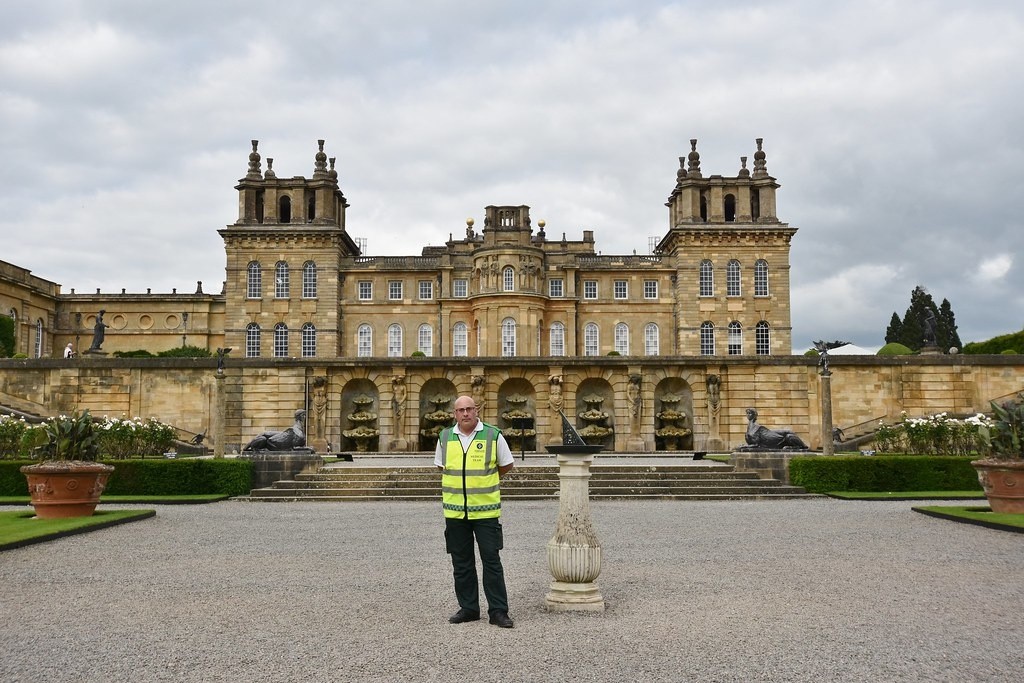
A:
[470,375,487,421]
[91,309,110,350]
[312,375,328,442]
[705,375,721,437]
[64,343,73,358]
[923,307,939,348]
[818,342,831,370]
[434,396,515,628]
[547,374,563,437]
[391,376,407,440]
[217,349,226,368]
[626,376,642,438]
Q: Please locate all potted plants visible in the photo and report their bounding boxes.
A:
[971,392,1024,514]
[20,407,114,519]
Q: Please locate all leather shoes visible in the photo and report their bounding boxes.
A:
[449,608,480,623]
[488,614,513,627]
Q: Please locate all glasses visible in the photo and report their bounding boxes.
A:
[456,406,475,412]
